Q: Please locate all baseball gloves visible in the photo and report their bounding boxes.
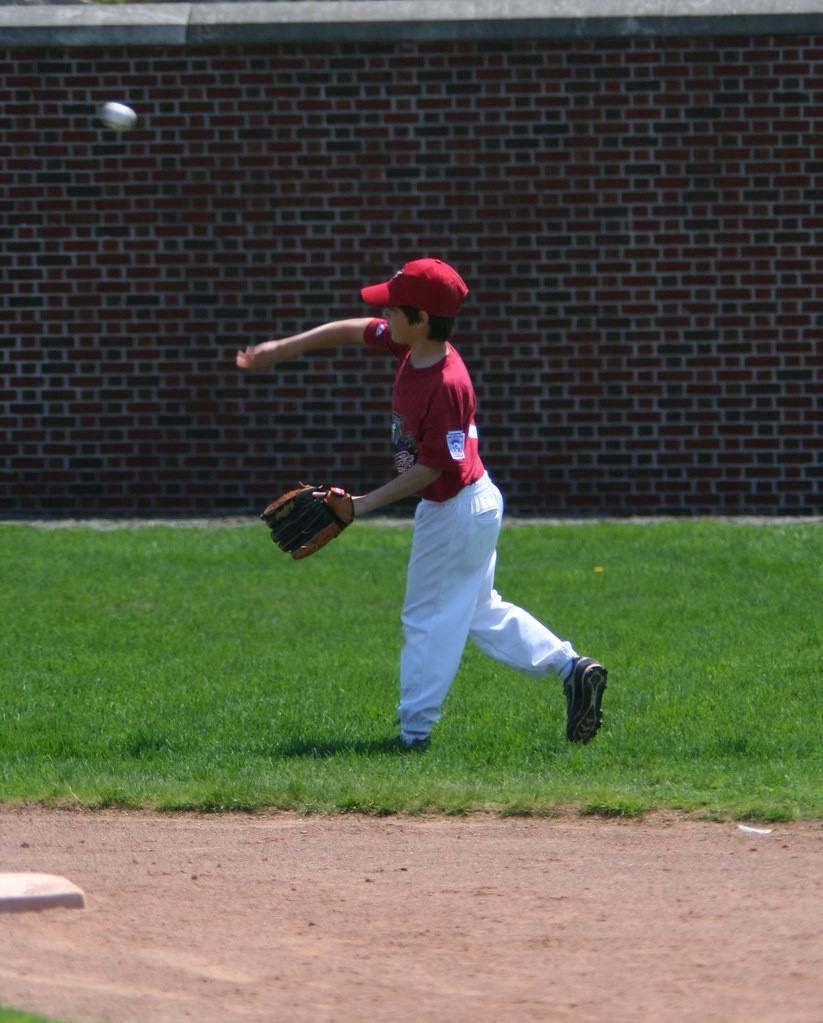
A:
[259,481,355,560]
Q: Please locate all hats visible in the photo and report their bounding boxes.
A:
[361,258,468,317]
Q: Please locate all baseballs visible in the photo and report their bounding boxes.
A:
[100,101,138,132]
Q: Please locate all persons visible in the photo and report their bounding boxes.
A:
[237,260,609,754]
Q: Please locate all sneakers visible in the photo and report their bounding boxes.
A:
[564,655,609,743]
[392,734,431,749]
